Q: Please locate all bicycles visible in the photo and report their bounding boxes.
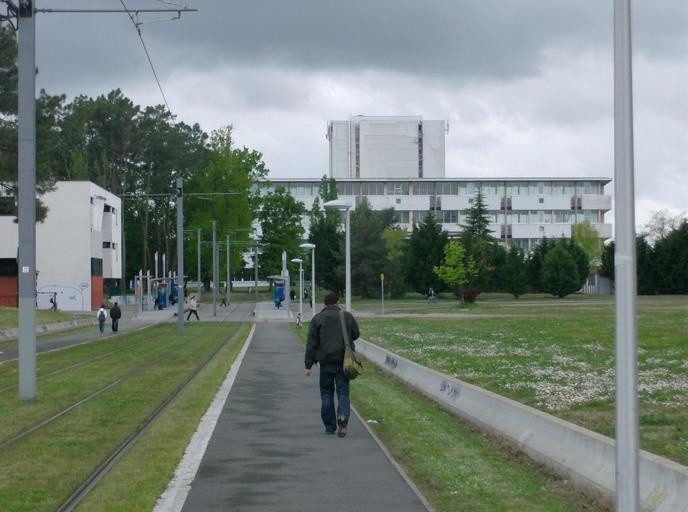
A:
[295,313,301,329]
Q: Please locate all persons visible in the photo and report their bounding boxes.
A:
[187,295,200,320]
[296,314,302,328]
[49,292,58,311]
[110,302,121,331]
[96,304,108,333]
[427,288,434,304]
[290,288,295,300]
[304,292,360,438]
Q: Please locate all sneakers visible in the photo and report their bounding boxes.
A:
[338,415,348,438]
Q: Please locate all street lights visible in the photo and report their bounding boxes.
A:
[322,199,351,312]
[195,228,203,282]
[290,259,304,319]
[224,232,232,305]
[298,243,315,322]
[209,220,218,315]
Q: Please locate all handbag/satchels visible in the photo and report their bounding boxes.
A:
[344,348,362,379]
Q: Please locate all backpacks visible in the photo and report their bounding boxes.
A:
[99,311,105,322]
